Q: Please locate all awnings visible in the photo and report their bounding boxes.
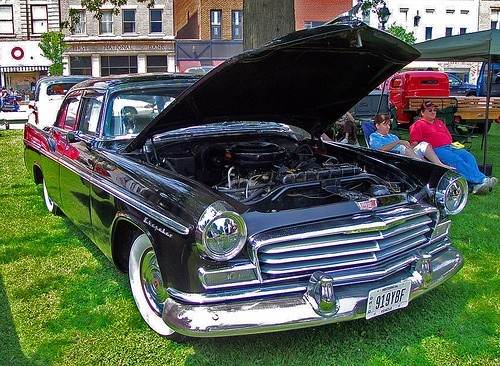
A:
[179,61,201,71]
[0,41,54,72]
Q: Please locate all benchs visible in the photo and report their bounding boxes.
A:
[65,114,152,135]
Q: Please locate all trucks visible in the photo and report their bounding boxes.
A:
[378,61,500,134]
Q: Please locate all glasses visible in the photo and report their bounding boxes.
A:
[425,110,436,112]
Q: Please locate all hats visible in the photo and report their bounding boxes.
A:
[421,101,439,109]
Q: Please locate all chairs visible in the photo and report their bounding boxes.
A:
[359,105,480,152]
[119,106,140,135]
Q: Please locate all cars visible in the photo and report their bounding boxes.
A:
[24,1,469,341]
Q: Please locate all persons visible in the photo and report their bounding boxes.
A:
[322,111,359,145]
[369,114,456,170]
[0,87,13,108]
[410,101,498,195]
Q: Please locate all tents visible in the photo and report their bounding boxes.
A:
[377,29,500,175]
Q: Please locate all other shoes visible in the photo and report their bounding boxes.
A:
[472,184,489,195]
[483,177,498,192]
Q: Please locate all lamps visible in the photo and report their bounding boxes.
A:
[192,46,196,54]
